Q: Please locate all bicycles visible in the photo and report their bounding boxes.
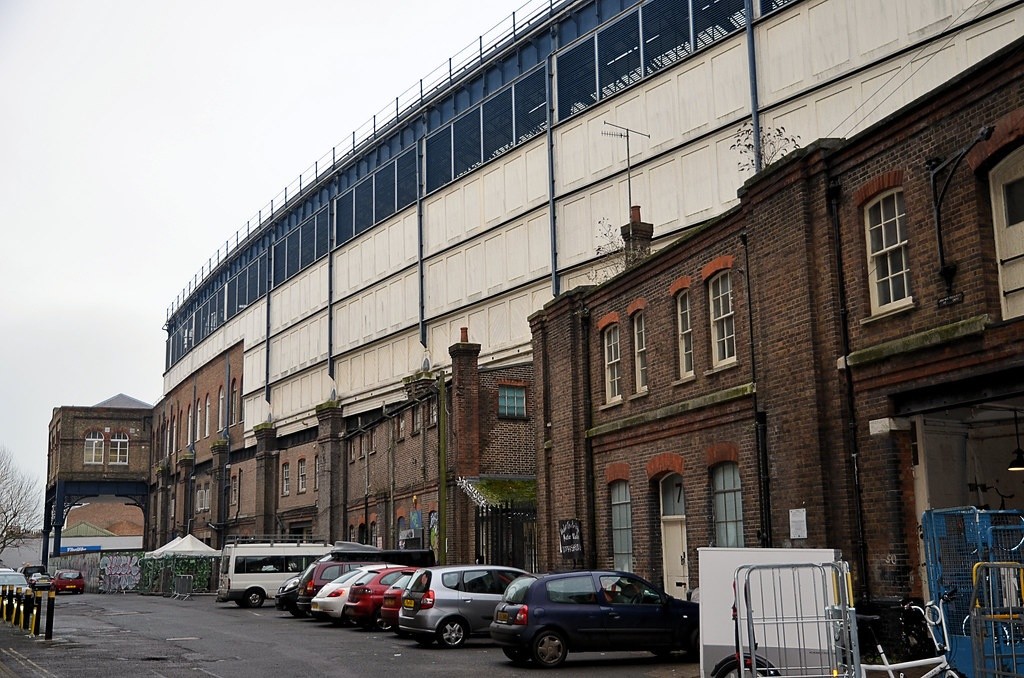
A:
[836,587,964,678]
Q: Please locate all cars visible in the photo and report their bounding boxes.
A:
[490,569,700,670]
[28,573,52,590]
[381,569,413,637]
[51,569,83,595]
[0,572,36,603]
[396,565,532,649]
[344,566,405,628]
[18,564,47,584]
[274,568,305,619]
[0,568,14,580]
[310,562,409,622]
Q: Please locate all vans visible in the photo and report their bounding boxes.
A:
[216,534,334,611]
[296,549,433,617]
[306,561,389,598]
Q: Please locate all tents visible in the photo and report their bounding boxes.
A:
[137,534,224,596]
